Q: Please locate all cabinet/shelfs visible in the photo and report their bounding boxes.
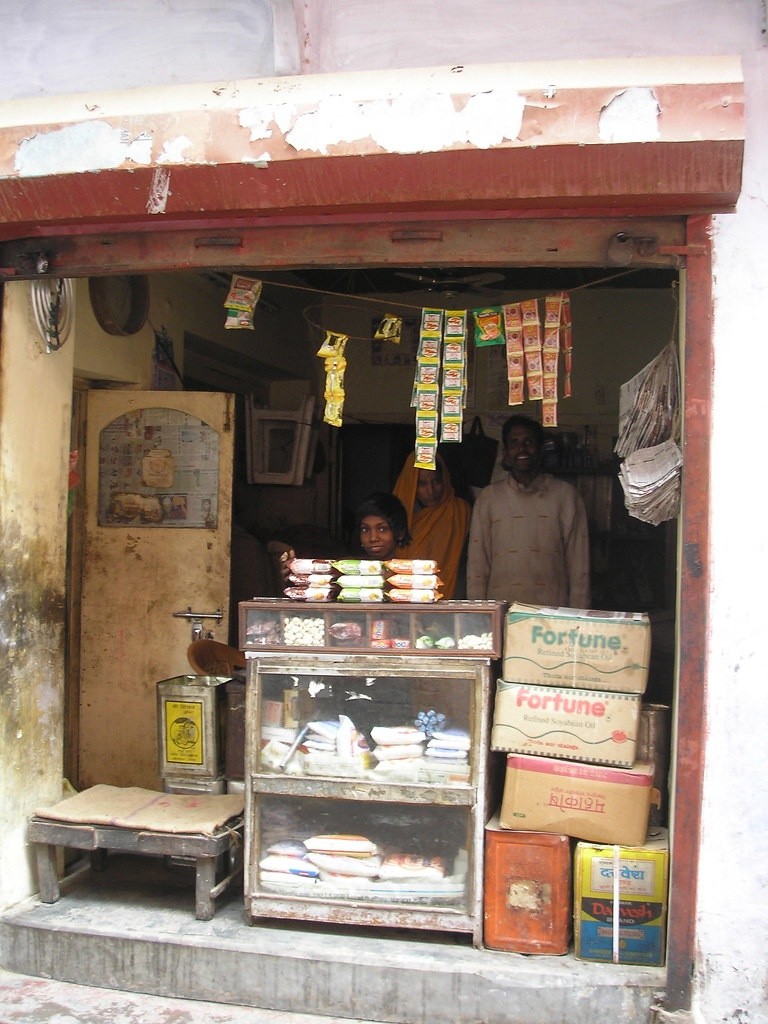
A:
[243,653,490,953]
[238,599,506,661]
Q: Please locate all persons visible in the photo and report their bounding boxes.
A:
[277,492,412,588]
[465,414,591,609]
[392,449,472,600]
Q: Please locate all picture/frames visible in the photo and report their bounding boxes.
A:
[370,316,421,365]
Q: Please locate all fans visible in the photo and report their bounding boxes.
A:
[395,272,505,298]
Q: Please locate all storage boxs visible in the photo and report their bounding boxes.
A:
[482,809,571,956]
[500,753,655,848]
[490,678,642,768]
[574,829,668,967]
[502,602,652,695]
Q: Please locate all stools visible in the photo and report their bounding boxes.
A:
[243,392,323,488]
[29,784,244,921]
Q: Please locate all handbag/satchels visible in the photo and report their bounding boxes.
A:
[453,416,500,487]
